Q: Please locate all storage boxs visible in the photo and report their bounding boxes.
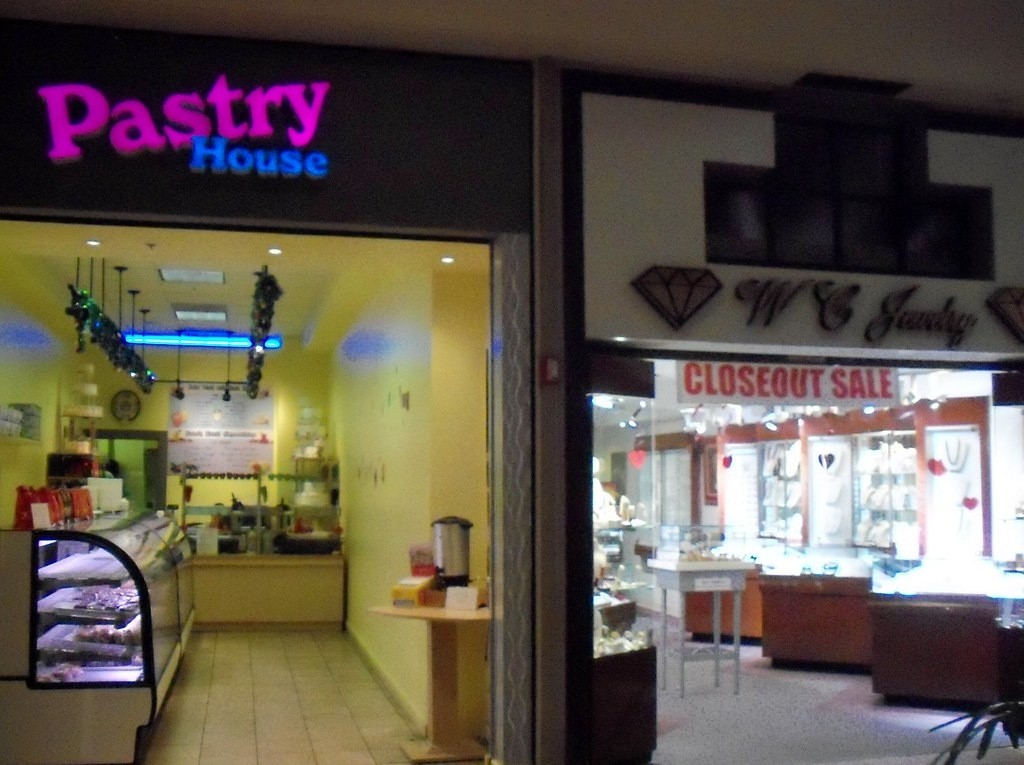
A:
[21,426,40,441]
[21,415,41,429]
[392,575,433,609]
[9,402,42,417]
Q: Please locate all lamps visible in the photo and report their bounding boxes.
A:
[172,329,185,400]
[245,265,283,399]
[65,257,156,394]
[222,331,235,402]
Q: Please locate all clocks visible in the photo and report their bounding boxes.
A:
[112,390,141,422]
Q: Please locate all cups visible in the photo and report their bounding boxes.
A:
[81,478,123,513]
[196,527,218,555]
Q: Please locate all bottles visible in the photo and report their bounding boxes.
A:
[247,525,257,557]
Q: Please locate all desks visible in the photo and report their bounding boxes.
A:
[368,604,492,762]
